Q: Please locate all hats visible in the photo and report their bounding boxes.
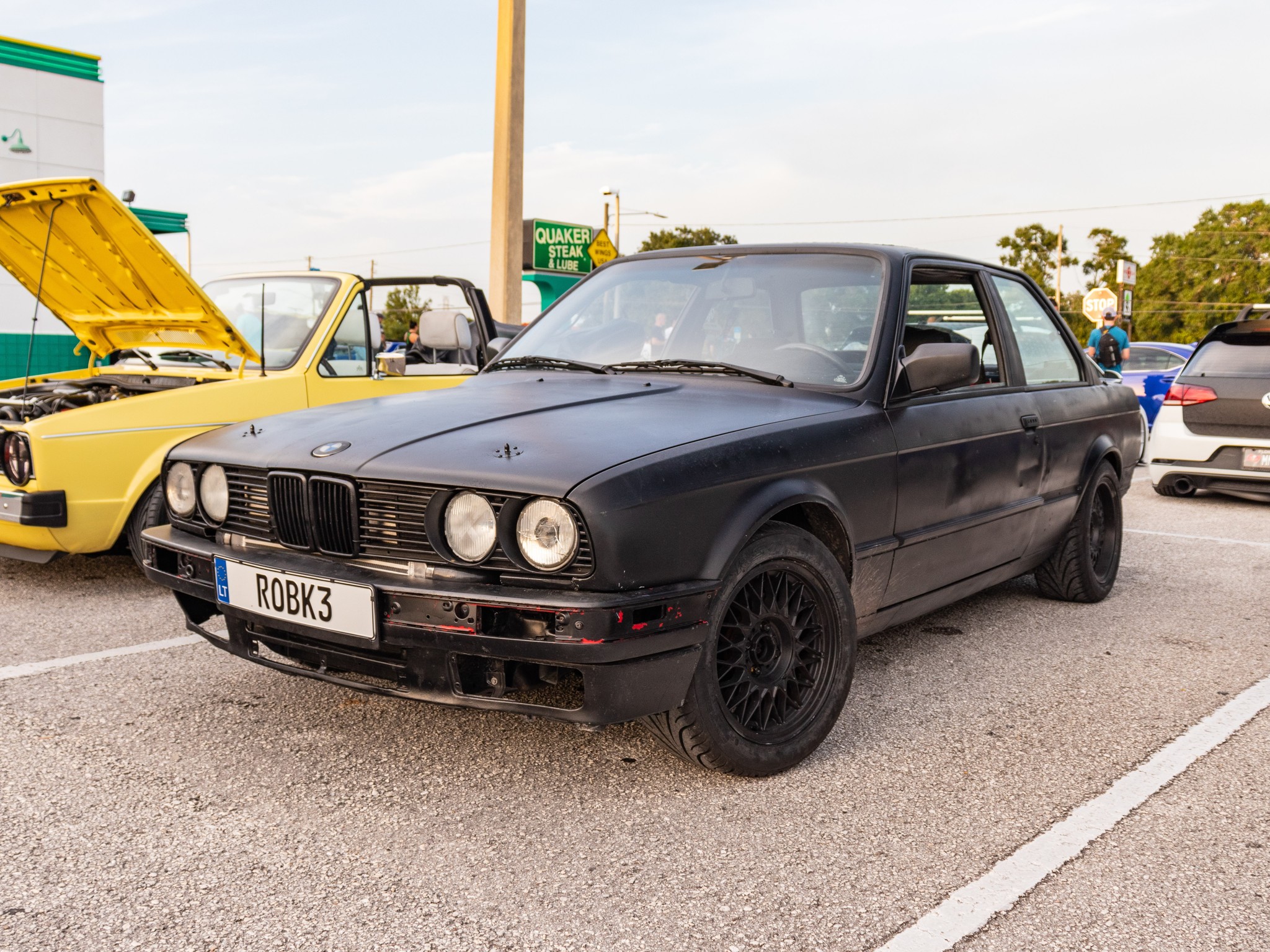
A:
[1101,307,1117,317]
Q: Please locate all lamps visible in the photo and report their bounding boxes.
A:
[0,127,32,155]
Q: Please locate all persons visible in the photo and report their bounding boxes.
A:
[569,301,744,368]
[1086,307,1131,376]
[373,312,387,366]
[403,321,420,352]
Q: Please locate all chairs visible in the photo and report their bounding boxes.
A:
[400,305,480,377]
[319,306,385,378]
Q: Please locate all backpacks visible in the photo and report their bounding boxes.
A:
[1099,326,1122,367]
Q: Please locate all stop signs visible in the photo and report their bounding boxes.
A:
[1082,287,1118,323]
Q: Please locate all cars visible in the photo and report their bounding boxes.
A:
[1083,341,1199,434]
[0,174,528,579]
[952,323,1068,365]
[139,243,1142,776]
[1147,303,1270,505]
